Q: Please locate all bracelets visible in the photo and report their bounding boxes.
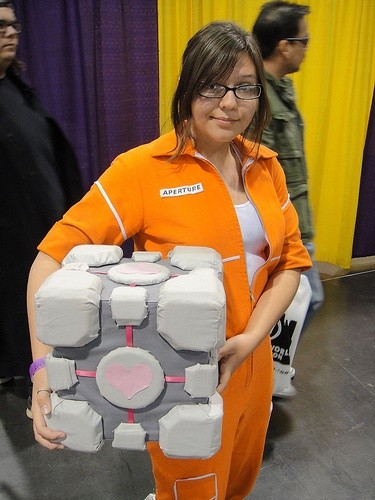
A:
[28,357,51,383]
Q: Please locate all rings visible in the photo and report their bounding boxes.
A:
[36,388,51,397]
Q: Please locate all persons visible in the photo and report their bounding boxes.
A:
[242,0,324,397]
[26,23,314,500]
[0,0,86,419]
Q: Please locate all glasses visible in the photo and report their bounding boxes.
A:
[286,33,309,47]
[195,81,264,99]
[0,19,21,32]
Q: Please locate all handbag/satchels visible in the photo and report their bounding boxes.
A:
[265,275,313,390]
